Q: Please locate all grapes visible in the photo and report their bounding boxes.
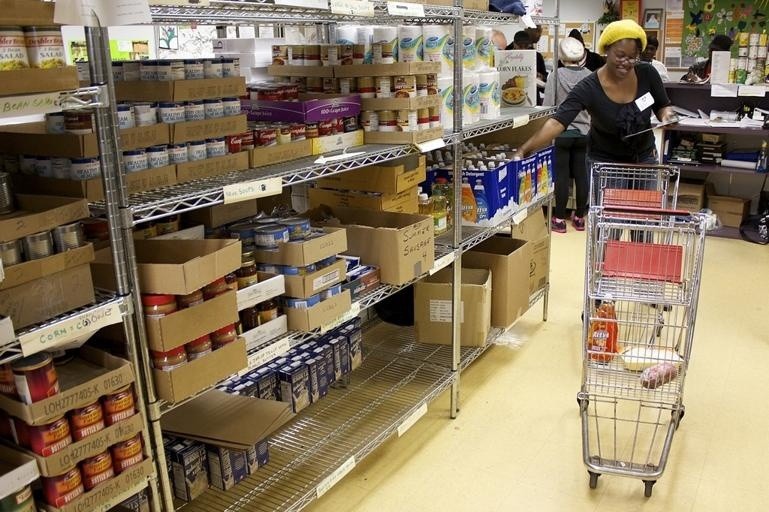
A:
[502,75,521,90]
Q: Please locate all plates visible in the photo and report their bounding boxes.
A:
[501,88,527,107]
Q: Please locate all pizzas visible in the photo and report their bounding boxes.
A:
[502,88,526,104]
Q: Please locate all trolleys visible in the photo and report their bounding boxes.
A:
[578,205,716,499]
[582,160,683,336]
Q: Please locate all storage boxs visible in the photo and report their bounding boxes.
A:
[1,0,561,512]
[666,171,709,215]
[705,181,752,229]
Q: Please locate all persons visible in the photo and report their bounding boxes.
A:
[680,34,732,85]
[485,24,671,233]
[512,19,674,312]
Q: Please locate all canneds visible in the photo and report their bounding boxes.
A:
[0,25,440,512]
[729,32,769,84]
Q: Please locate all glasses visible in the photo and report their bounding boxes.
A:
[616,53,640,64]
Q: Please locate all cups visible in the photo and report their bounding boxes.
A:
[515,78,525,88]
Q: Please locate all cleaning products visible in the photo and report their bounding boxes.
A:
[518,155,552,207]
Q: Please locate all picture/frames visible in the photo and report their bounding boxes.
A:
[642,7,663,29]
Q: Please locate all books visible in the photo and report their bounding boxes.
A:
[671,118,765,170]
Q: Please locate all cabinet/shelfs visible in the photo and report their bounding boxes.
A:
[658,81,769,176]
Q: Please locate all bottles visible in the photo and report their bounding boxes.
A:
[520,157,553,207]
[418,178,451,238]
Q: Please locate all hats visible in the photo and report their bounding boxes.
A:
[559,37,588,69]
[598,20,648,57]
[514,30,529,44]
[710,35,732,50]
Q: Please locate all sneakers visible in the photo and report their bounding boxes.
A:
[552,216,567,233]
[571,214,585,231]
